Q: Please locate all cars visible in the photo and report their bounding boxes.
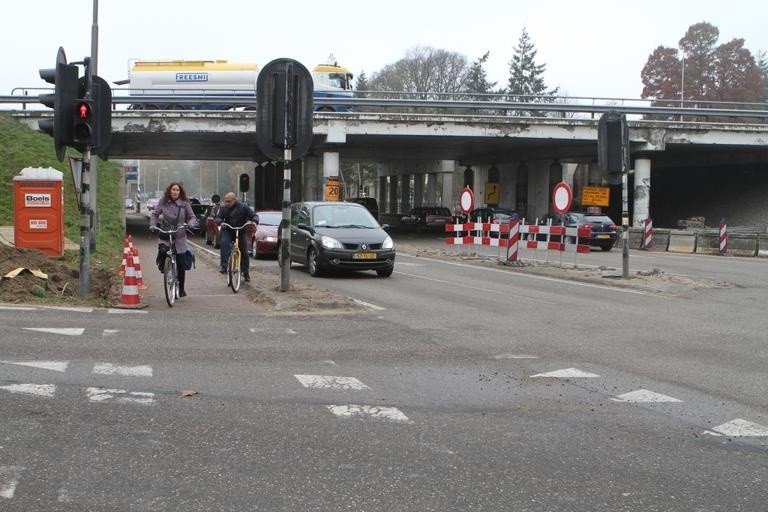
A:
[530,211,618,252]
[146,197,228,248]
[125,198,134,209]
[246,210,285,259]
[459,207,523,237]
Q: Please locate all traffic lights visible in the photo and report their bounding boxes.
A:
[71,93,99,150]
[239,173,249,193]
[36,67,55,138]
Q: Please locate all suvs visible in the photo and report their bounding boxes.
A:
[399,206,453,234]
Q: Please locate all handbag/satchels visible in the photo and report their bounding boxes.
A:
[184,245,196,270]
[157,223,178,241]
[156,242,170,274]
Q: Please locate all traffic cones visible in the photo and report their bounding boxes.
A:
[127,234,135,253]
[118,238,133,276]
[113,255,150,310]
[129,247,148,291]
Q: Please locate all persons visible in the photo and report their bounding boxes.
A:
[149,182,197,297]
[215,192,259,282]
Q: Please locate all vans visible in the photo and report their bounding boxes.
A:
[344,197,378,222]
[275,200,397,278]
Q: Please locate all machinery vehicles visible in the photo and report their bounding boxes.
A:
[128,58,355,113]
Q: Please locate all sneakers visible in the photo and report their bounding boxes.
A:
[219,266,227,273]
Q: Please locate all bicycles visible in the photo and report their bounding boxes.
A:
[149,223,201,307]
[219,221,254,293]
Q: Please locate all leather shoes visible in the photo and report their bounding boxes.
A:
[242,272,250,282]
[179,291,187,297]
